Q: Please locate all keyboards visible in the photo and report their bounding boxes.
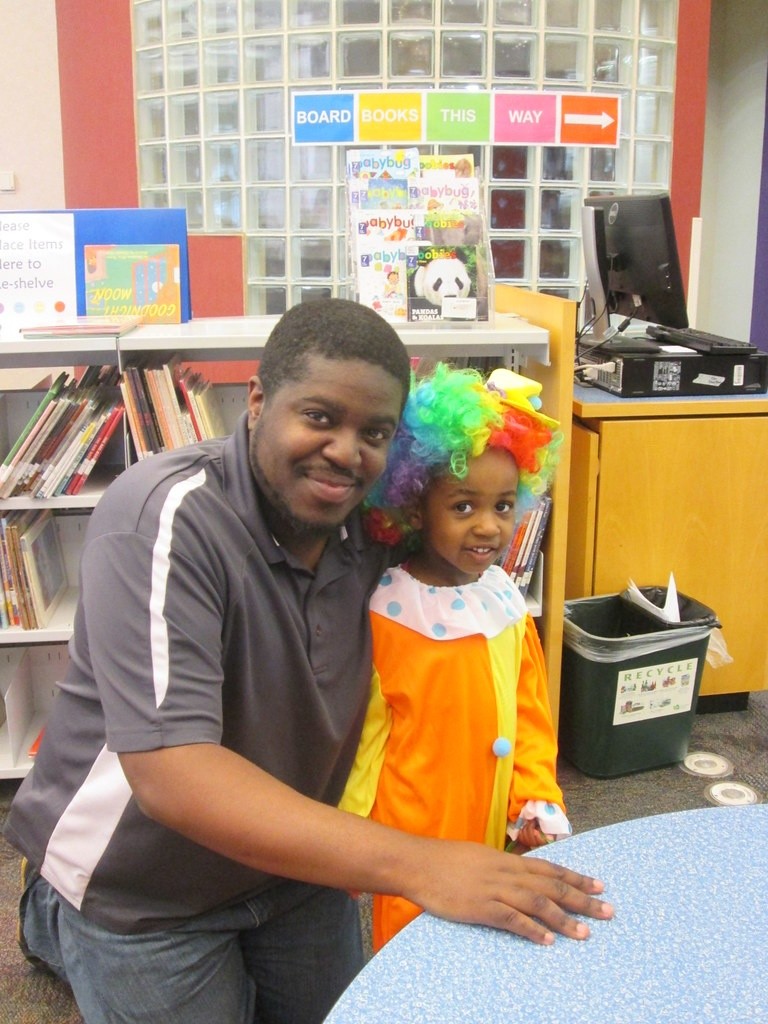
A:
[645,325,758,354]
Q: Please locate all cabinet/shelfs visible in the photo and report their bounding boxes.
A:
[1,308,555,781]
[488,280,768,716]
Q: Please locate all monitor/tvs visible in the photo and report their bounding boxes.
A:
[584,191,689,331]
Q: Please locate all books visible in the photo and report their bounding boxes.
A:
[1,365,234,500]
[1,510,70,630]
[341,148,498,322]
[444,352,552,609]
[15,312,143,337]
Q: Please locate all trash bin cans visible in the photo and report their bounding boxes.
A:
[561,584,721,781]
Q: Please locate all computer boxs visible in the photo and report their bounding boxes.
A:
[574,331,768,398]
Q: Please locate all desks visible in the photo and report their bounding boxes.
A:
[315,804,768,1024]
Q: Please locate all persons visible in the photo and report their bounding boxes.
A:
[333,433,583,963]
[0,296,619,1024]
